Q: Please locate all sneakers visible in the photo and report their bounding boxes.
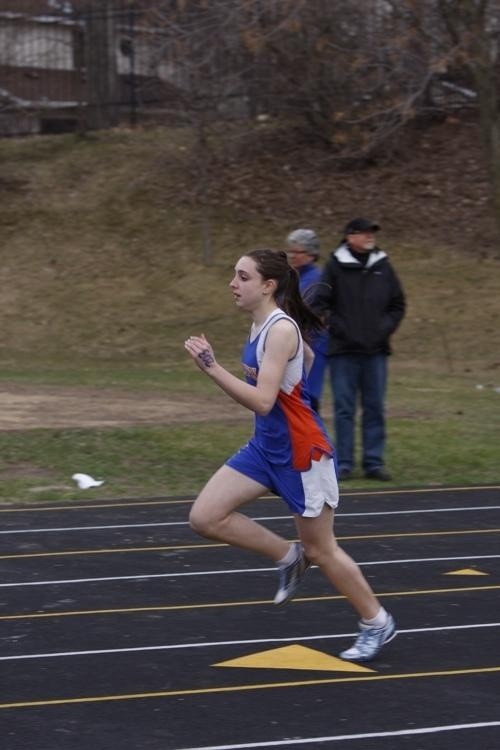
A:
[339,614,396,662]
[370,468,391,481]
[337,470,348,480]
[272,543,311,608]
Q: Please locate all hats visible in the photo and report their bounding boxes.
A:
[346,217,382,234]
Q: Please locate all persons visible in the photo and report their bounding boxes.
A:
[319,217,409,481]
[284,226,329,415]
[184,246,398,661]
